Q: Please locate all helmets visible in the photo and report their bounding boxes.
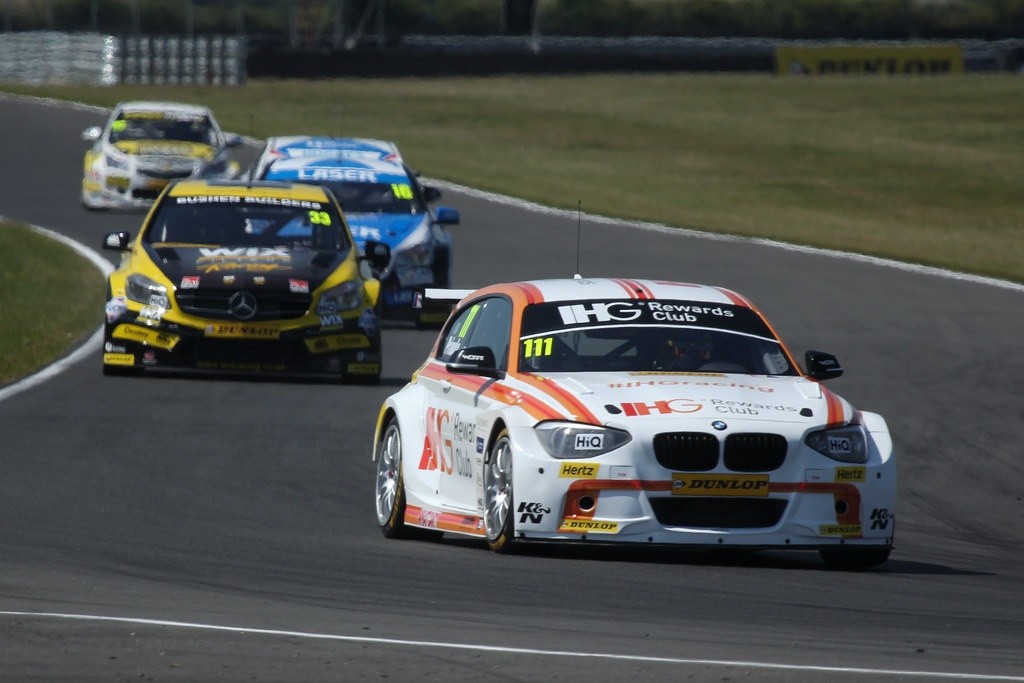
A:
[666,329,712,364]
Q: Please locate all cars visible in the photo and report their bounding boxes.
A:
[78,102,247,212]
[252,156,459,330]
[101,179,385,386]
[376,270,901,572]
[240,133,440,202]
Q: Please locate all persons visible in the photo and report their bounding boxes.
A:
[648,328,718,371]
